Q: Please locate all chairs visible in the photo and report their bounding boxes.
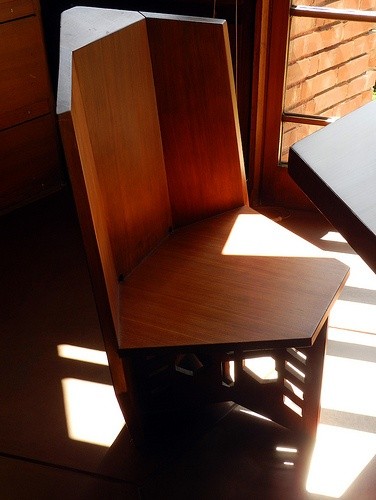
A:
[55,4,355,444]
